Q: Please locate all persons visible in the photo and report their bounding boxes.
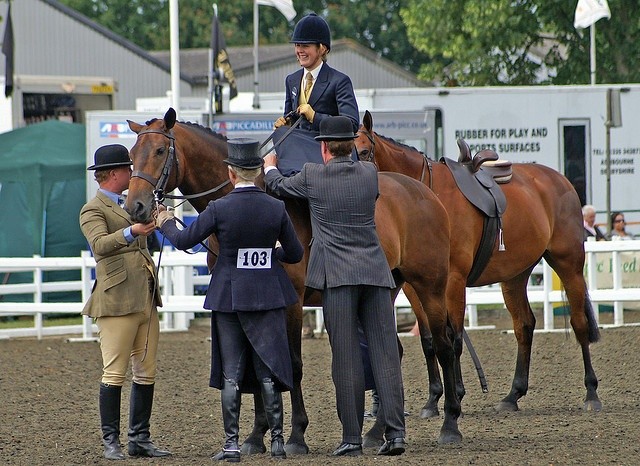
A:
[274,11,359,135]
[261,116,408,457]
[77,144,174,460]
[582,205,607,241]
[157,136,305,462]
[605,213,634,240]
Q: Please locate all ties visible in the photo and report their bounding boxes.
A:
[304,72,313,100]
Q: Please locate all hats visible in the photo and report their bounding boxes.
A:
[224,137,265,170]
[289,13,331,53]
[314,116,359,139]
[86,144,134,169]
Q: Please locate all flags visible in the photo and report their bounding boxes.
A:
[211,10,238,113]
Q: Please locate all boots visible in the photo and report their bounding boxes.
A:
[129,383,172,457]
[260,377,286,460]
[212,372,241,462]
[99,381,128,460]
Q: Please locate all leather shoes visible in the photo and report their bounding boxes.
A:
[377,438,405,455]
[332,442,363,457]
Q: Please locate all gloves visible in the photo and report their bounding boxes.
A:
[156,210,174,229]
[274,116,292,128]
[297,103,315,124]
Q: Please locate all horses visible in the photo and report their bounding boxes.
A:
[352,110,606,415]
[123,107,463,454]
